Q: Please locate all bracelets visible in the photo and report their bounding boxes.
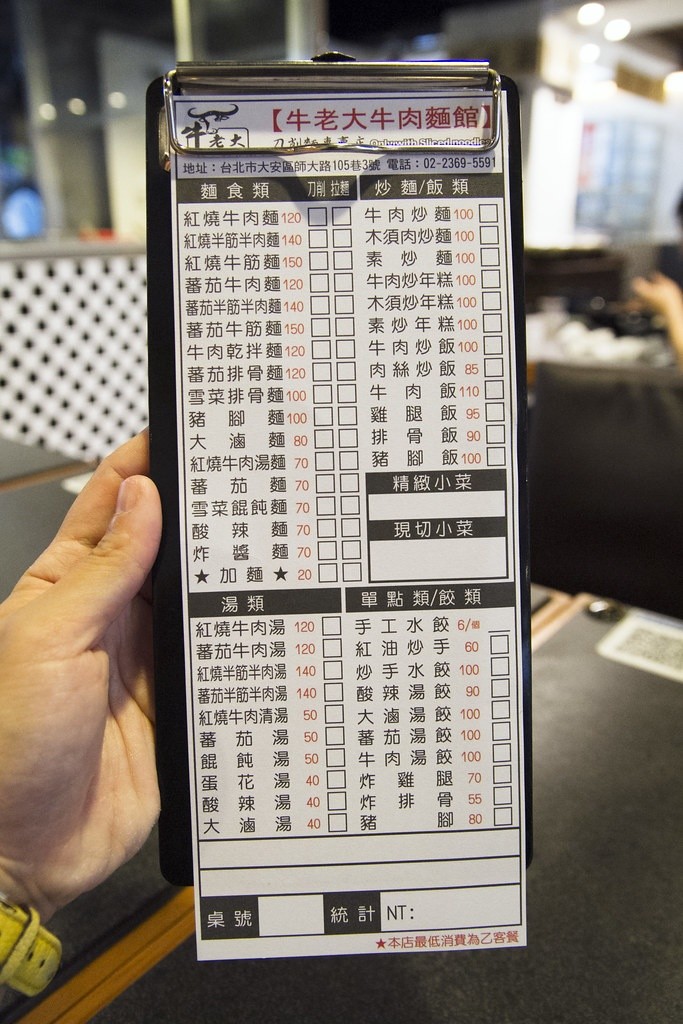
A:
[0,893,63,998]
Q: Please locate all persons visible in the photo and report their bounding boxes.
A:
[633,198,683,369]
[0,428,163,1024]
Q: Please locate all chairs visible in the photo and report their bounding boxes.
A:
[527,356,683,605]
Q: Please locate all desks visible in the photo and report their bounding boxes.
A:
[0,432,683,1024]
